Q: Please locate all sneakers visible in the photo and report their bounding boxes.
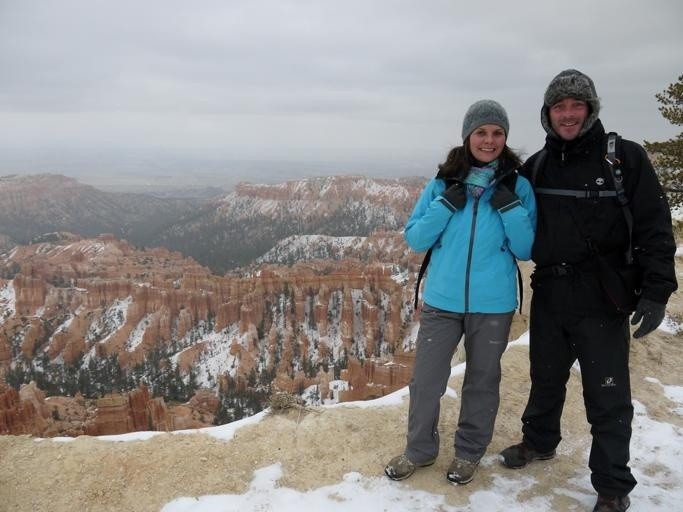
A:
[592,493,630,511]
[446,446,486,484]
[497,441,556,469]
[384,453,435,480]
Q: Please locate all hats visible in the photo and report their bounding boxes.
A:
[461,100,509,145]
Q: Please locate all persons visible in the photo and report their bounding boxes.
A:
[495,69,677,512]
[383,97,538,487]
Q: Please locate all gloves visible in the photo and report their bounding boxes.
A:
[441,182,465,209]
[489,182,521,212]
[630,297,665,338]
